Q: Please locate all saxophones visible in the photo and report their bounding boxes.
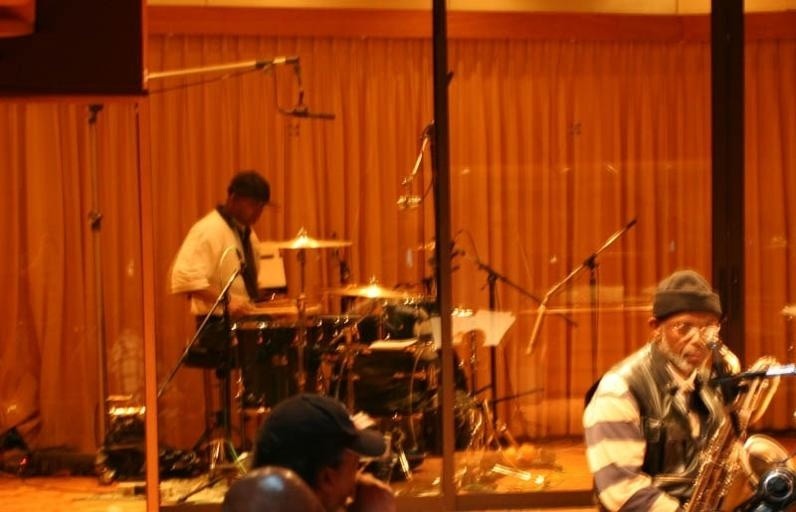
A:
[679,341,796,512]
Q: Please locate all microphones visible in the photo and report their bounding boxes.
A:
[699,326,722,351]
[279,107,336,119]
[397,195,421,208]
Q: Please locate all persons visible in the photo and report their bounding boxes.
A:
[582,267,742,511]
[252,392,397,511]
[220,464,326,511]
[168,169,280,367]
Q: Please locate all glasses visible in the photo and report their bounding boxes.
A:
[672,324,722,340]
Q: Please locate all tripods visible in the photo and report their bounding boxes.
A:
[157,245,248,503]
[435,258,549,487]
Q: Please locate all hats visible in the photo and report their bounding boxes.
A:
[653,271,722,320]
[228,172,279,209]
[252,394,386,470]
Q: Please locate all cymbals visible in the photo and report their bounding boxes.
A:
[331,281,417,304]
[264,234,353,250]
